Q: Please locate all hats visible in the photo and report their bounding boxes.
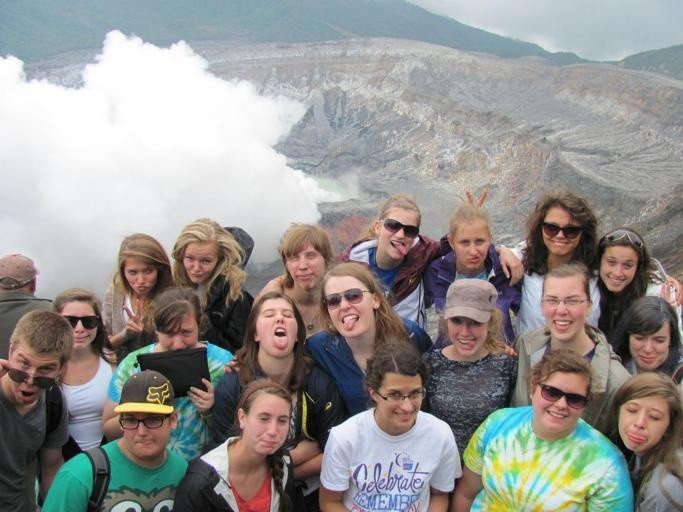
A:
[0,254,40,290]
[112,369,176,415]
[443,278,498,323]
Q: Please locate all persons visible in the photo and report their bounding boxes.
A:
[0,189,683,511]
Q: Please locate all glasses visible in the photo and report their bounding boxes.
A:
[374,386,426,402]
[542,297,589,307]
[118,413,172,429]
[538,382,592,410]
[322,287,373,310]
[380,219,420,239]
[603,229,647,253]
[3,365,58,389]
[63,315,101,330]
[543,221,584,239]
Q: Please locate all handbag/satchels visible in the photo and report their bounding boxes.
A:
[135,347,212,398]
